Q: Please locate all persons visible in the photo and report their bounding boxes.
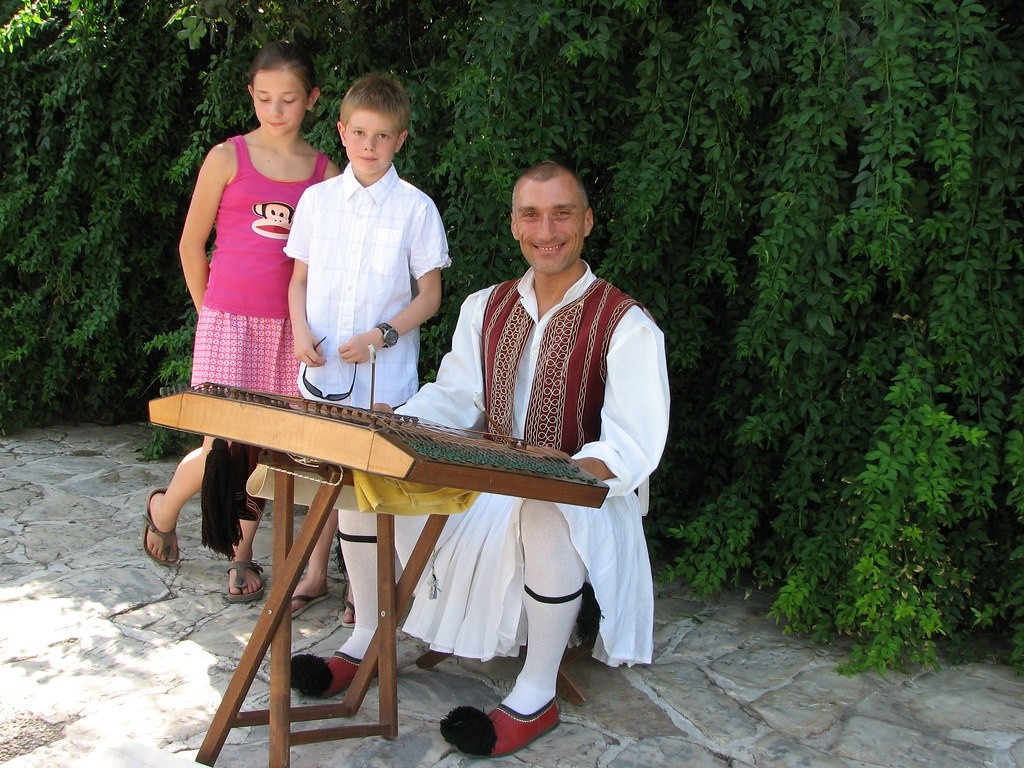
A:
[142,43,341,600]
[281,75,447,628]
[295,167,670,754]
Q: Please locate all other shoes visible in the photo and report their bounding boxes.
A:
[290,649,363,699]
[440,697,559,757]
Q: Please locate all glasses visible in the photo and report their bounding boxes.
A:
[302,336,357,401]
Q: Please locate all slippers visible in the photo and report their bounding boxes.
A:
[227,562,264,602]
[340,600,357,628]
[291,591,332,618]
[142,488,179,566]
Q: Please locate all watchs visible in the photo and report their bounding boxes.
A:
[376,322,398,348]
